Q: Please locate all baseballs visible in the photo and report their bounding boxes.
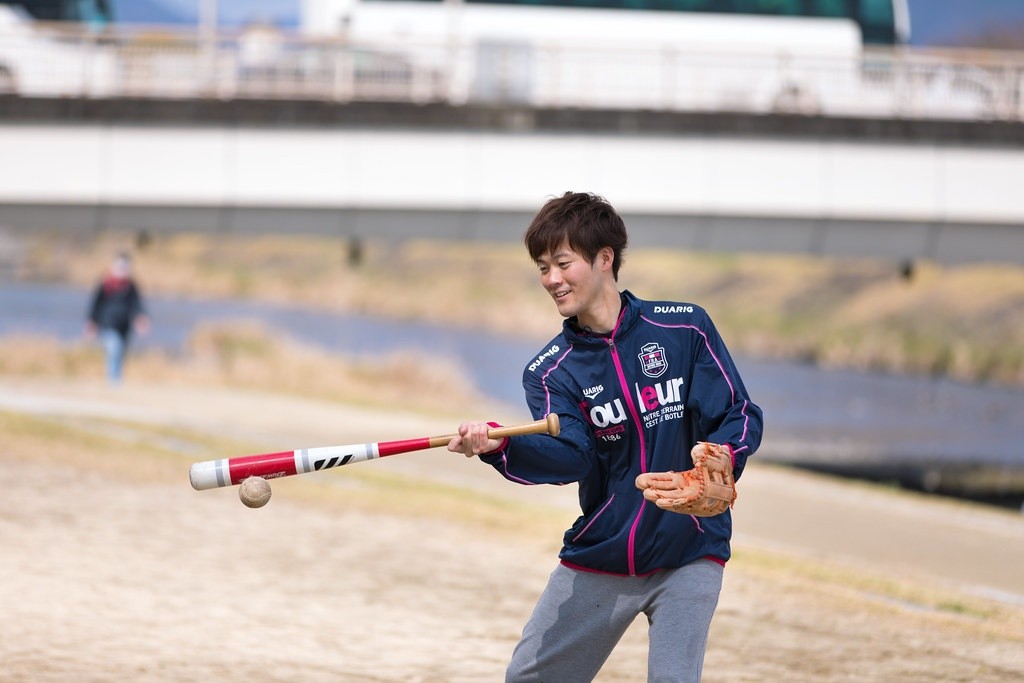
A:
[238,475,272,509]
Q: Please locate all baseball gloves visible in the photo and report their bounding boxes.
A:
[634,440,737,519]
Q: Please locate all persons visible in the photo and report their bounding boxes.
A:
[85,252,146,387]
[447,191,764,683]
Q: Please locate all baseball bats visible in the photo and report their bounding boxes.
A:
[188,413,561,491]
